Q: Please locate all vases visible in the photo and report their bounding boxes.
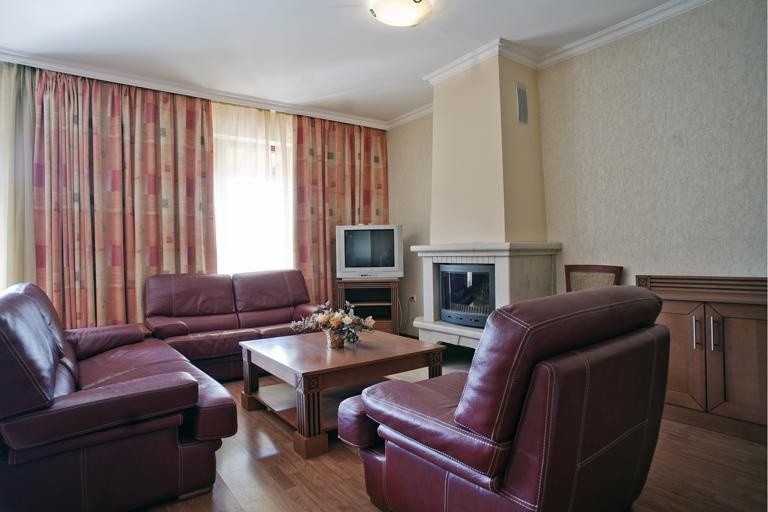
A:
[326,330,343,349]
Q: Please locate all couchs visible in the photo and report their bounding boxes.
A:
[0,282,237,512]
[338,287,671,512]
[145,269,320,382]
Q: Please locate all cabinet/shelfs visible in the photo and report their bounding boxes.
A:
[335,279,400,333]
[638,276,768,427]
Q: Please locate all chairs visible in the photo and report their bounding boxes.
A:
[565,265,623,291]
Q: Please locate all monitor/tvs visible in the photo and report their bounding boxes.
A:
[335,224,404,281]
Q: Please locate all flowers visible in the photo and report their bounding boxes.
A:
[290,302,378,343]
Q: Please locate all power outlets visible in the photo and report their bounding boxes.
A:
[410,295,416,301]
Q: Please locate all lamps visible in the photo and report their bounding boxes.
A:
[368,0,433,29]
